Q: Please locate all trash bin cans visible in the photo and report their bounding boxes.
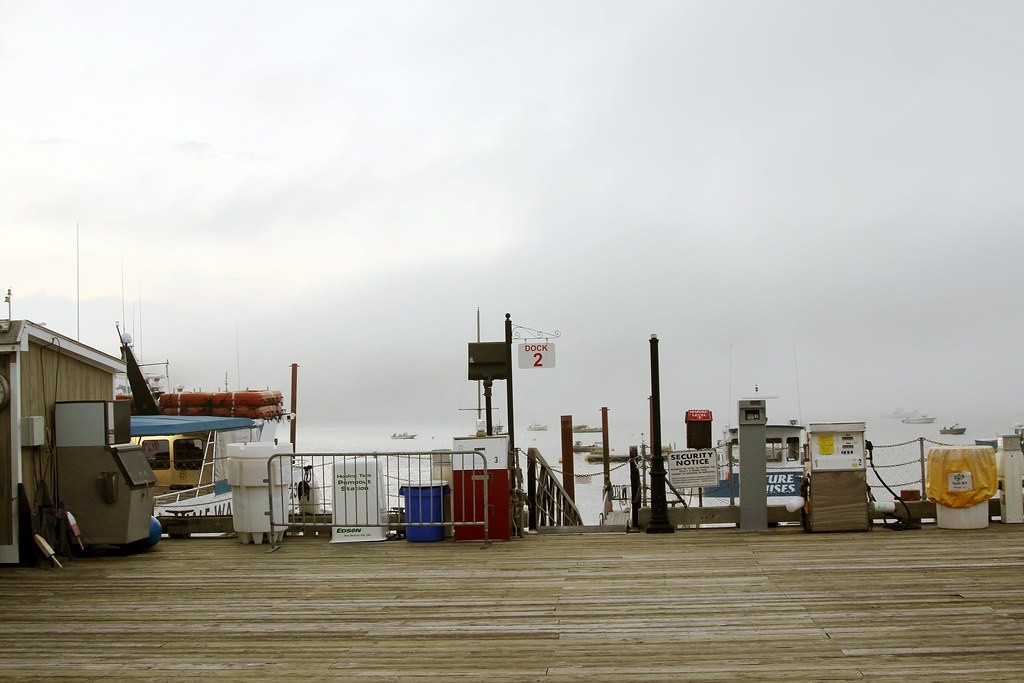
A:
[928,446,995,529]
[399,481,451,542]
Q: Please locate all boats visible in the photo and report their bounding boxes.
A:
[115,319,1000,538]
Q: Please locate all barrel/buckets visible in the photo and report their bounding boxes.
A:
[401,481,450,542]
